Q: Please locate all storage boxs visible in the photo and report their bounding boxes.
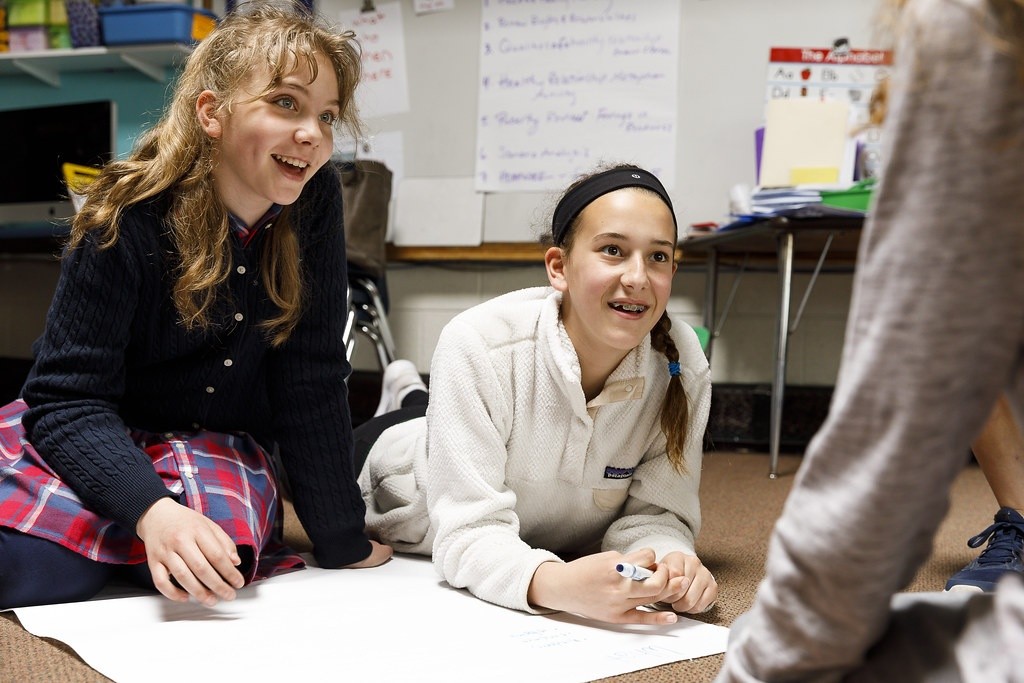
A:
[98,4,219,47]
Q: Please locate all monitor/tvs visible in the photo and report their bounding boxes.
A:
[0,99,117,223]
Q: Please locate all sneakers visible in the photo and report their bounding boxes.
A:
[372,358,430,418]
[940,505,1024,593]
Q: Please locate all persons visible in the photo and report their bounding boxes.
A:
[353,162,718,626]
[0,0,393,610]
[712,0,1023,683]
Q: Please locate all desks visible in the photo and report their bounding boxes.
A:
[678,207,865,479]
